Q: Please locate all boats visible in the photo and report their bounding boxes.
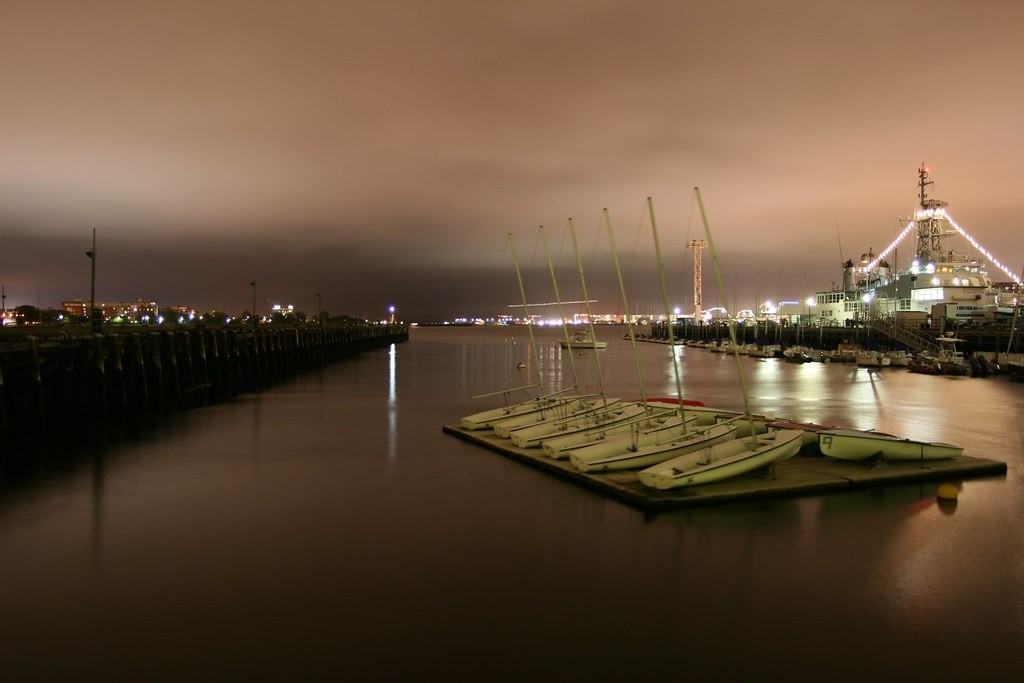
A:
[558,322,607,349]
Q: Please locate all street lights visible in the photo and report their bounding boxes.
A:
[250,280,256,326]
[317,295,324,327]
[86,227,98,320]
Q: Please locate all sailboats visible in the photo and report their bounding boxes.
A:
[443,179,1009,508]
